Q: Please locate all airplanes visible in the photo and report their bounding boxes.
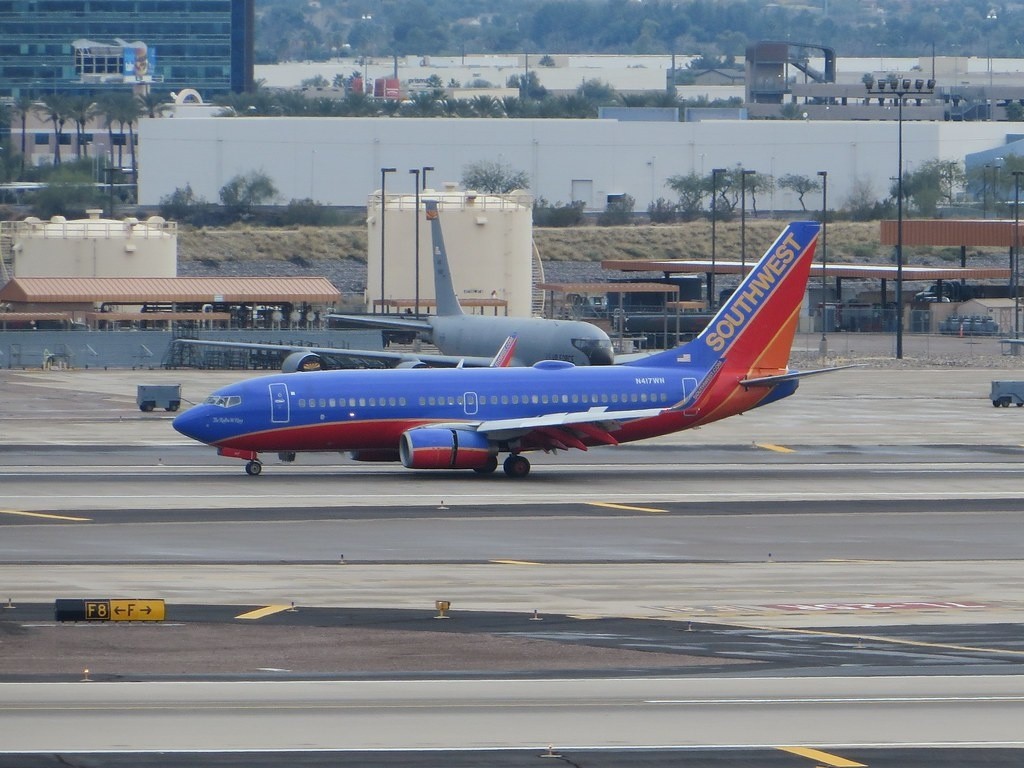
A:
[171,221,868,480]
[175,198,665,374]
